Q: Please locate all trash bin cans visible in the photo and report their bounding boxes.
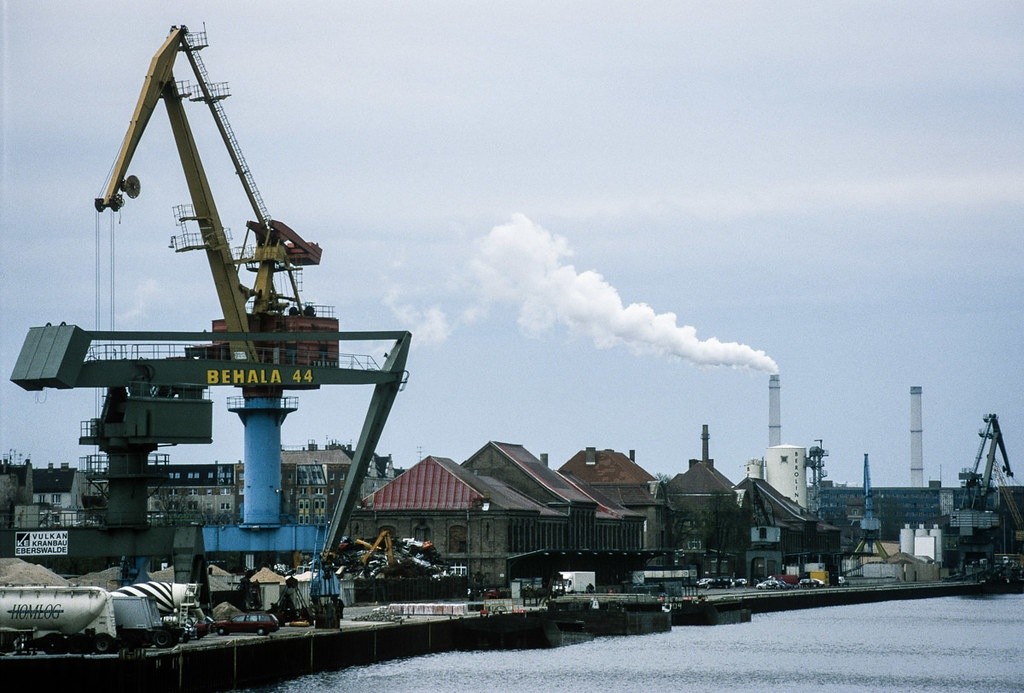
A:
[468,585,474,600]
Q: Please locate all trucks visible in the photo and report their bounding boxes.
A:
[798,571,830,587]
[551,571,596,594]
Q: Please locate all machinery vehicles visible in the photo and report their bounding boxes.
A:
[0,586,117,654]
[360,530,414,577]
[94,24,339,367]
[110,581,206,648]
[986,454,1024,532]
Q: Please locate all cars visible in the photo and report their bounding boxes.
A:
[484,588,501,599]
[209,611,281,635]
[756,580,780,590]
[696,575,747,589]
